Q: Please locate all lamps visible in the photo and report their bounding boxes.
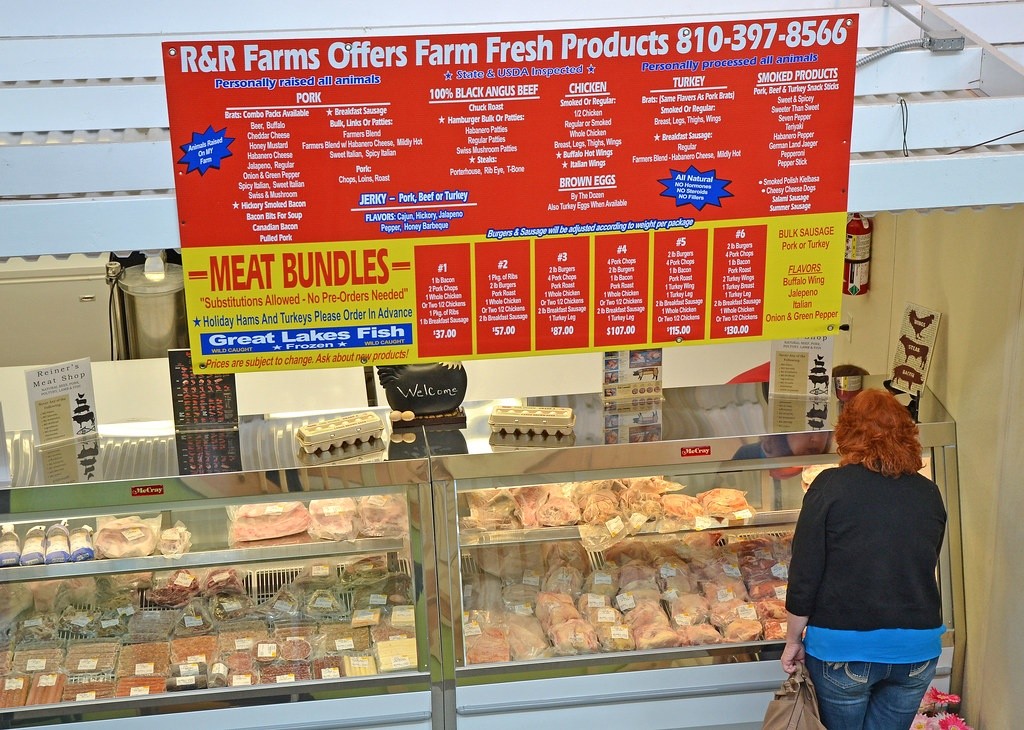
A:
[142,255,166,282]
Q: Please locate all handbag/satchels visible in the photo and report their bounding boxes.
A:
[761,660,827,730]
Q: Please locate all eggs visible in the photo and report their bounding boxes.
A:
[390,433,416,443]
[389,410,415,421]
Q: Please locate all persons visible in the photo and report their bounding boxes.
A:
[732,429,837,461]
[780,386,948,730]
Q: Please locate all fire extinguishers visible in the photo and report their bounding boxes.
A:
[842,212,873,296]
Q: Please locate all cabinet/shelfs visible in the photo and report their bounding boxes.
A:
[0,405,444,730]
[423,374,966,730]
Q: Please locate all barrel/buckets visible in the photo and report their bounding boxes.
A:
[111,261,189,360]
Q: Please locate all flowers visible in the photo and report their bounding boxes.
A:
[908,686,974,730]
